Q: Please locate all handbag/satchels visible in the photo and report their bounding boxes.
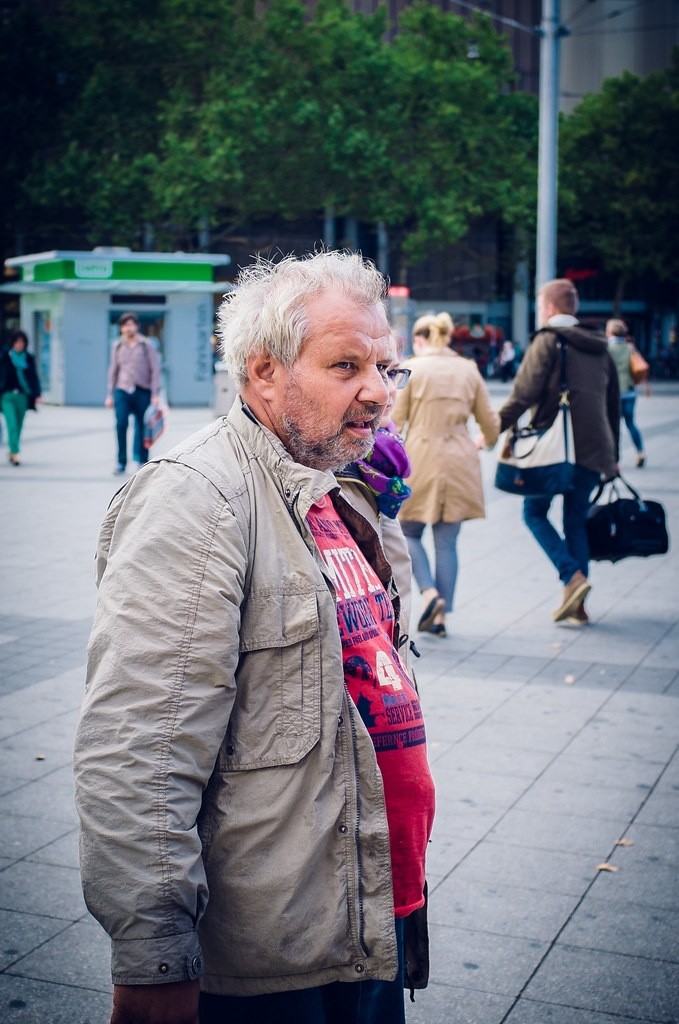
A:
[495,394,580,497]
[143,402,164,448]
[628,342,653,396]
[586,476,670,562]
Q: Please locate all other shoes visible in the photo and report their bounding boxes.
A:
[8,456,19,466]
[115,461,125,474]
[637,457,645,468]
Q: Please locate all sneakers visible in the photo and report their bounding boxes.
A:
[414,593,448,637]
[555,569,591,626]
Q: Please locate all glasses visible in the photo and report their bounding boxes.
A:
[391,366,412,387]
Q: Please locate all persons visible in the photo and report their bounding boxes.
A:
[105,313,159,476]
[605,317,648,467]
[74,250,436,1023]
[0,330,41,467]
[453,323,517,382]
[497,277,620,624]
[387,313,500,638]
